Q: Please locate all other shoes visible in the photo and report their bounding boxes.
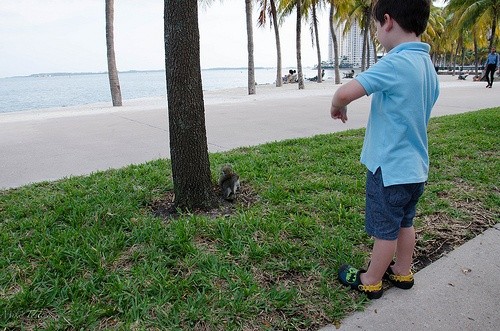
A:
[489,86,492,88]
[486,83,490,87]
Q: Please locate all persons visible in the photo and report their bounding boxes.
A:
[284,70,297,83]
[330,0,439,299]
[486,47,500,88]
[322,70,324,77]
[350,70,355,78]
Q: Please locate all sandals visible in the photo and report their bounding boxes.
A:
[368,259,414,289]
[339,265,383,299]
